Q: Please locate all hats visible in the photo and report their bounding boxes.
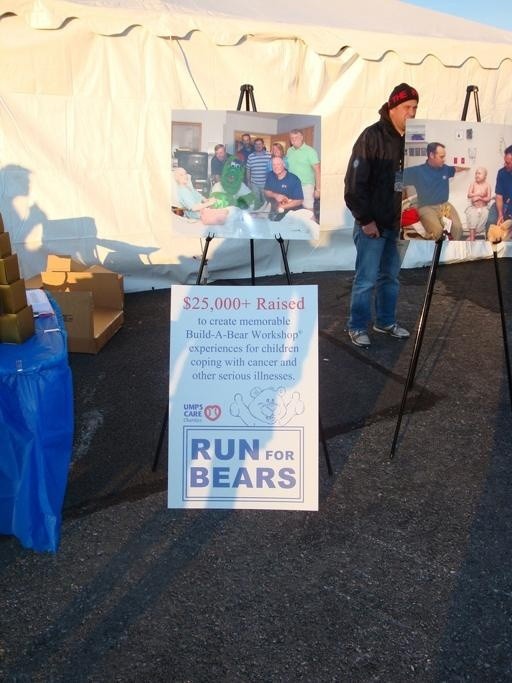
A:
[388,83,418,109]
[274,141,286,154]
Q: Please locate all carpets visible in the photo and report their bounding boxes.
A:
[1,289,434,548]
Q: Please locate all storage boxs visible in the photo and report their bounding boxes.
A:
[0,211,126,356]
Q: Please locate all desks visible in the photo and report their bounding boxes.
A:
[1,285,73,556]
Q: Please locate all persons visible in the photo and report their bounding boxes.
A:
[402,141,470,241]
[484,145,512,242]
[464,167,491,241]
[344,82,420,349]
[172,127,321,225]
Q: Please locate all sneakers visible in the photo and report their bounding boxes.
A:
[373,323,410,338]
[349,329,371,347]
